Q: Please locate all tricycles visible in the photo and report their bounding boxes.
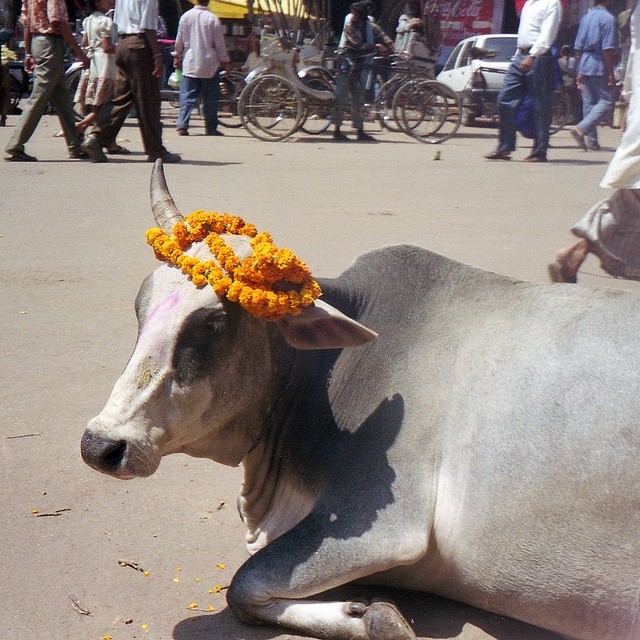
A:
[0,44,72,109]
[217,43,462,143]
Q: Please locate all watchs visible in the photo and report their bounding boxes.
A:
[530,54,535,58]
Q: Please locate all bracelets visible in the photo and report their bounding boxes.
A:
[25,53,31,59]
[153,53,162,59]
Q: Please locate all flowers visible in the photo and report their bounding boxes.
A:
[146,210,322,321]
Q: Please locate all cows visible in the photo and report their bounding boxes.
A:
[81,156,640,640]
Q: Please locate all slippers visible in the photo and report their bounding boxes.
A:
[3,153,36,161]
[523,156,546,161]
[485,152,511,161]
[547,263,575,284]
[570,129,587,151]
[68,151,83,159]
[589,144,599,150]
[107,146,131,154]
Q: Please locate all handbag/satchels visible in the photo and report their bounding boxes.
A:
[612,96,626,129]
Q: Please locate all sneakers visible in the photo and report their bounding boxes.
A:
[80,135,107,163]
[146,147,181,163]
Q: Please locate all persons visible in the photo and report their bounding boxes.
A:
[404,8,436,80]
[4,0,91,162]
[330,2,406,143]
[485,0,562,163]
[73,0,131,156]
[78,0,184,164]
[394,1,423,61]
[610,0,635,132]
[556,44,582,123]
[338,0,376,89]
[241,37,266,75]
[548,1,640,284]
[244,24,259,55]
[170,1,232,136]
[157,15,168,39]
[570,0,621,152]
[257,14,289,56]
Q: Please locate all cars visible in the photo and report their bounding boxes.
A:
[436,34,564,127]
